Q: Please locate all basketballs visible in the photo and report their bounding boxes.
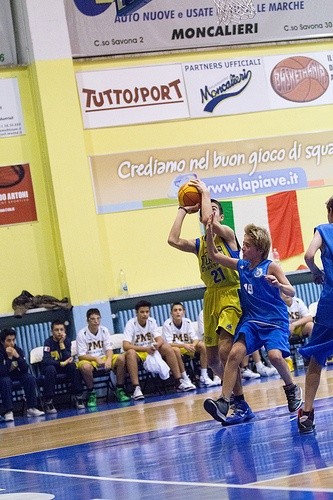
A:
[178,182,201,212]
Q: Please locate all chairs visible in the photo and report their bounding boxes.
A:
[0,301,318,420]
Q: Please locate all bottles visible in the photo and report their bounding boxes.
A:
[118,269,128,297]
[270,247,283,270]
[295,348,304,369]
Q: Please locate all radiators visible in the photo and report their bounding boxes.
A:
[0,321,73,402]
[111,282,323,381]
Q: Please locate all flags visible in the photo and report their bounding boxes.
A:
[199,190,304,262]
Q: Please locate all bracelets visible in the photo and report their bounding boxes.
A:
[178,207,188,213]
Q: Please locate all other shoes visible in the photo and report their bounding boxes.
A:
[74,393,85,408]
[258,364,276,376]
[45,398,57,414]
[198,373,218,387]
[131,385,145,400]
[212,374,222,385]
[4,411,14,421]
[27,408,45,417]
[269,362,278,374]
[240,366,260,379]
[183,375,197,390]
[176,378,195,392]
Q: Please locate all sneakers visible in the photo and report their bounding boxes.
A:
[222,399,255,426]
[203,397,229,422]
[116,387,130,401]
[297,408,316,433]
[283,383,303,412]
[86,389,97,406]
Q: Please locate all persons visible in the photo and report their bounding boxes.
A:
[279,289,314,340]
[197,309,254,385]
[203,210,302,422]
[309,302,318,323]
[297,196,333,434]
[168,179,254,426]
[241,351,276,381]
[77,307,130,407]
[162,302,217,390]
[0,327,45,421]
[123,300,193,400]
[39,319,85,414]
